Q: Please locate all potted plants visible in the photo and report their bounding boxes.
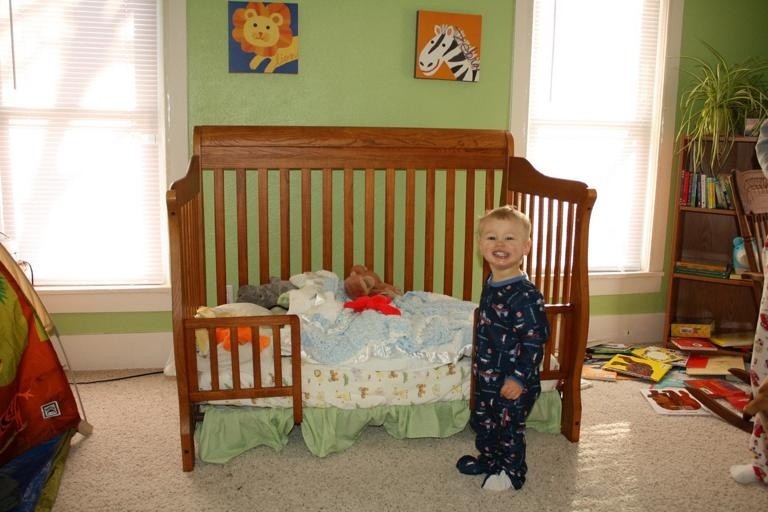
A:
[666,32,768,179]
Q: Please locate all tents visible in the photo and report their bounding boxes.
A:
[0,243,93,512]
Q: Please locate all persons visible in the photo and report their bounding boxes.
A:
[456,204,550,492]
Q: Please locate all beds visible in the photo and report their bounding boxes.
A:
[165,121,598,472]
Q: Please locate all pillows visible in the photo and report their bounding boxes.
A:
[194,302,275,357]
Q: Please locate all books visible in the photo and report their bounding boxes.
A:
[584,331,755,400]
[680,167,735,209]
[675,260,765,282]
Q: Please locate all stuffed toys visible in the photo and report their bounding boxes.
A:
[345,265,400,297]
[240,276,298,308]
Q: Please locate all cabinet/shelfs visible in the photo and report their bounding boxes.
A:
[663,131,768,356]
